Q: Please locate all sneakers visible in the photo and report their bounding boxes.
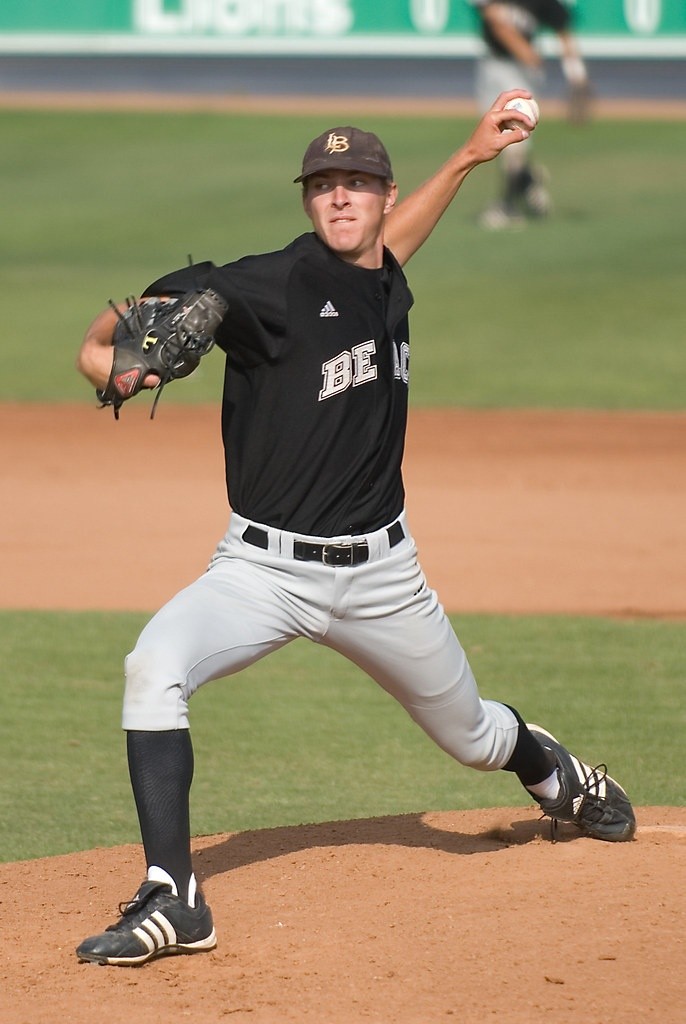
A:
[520,723,637,845]
[76,881,216,965]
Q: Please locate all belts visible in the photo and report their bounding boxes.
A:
[241,520,405,569]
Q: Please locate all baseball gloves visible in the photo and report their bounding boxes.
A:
[95,286,228,419]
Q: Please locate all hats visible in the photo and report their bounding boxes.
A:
[294,126,393,188]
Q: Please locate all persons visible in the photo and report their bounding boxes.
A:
[478,1,587,224]
[74,91,635,969]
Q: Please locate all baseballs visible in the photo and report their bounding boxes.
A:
[504,96,538,134]
